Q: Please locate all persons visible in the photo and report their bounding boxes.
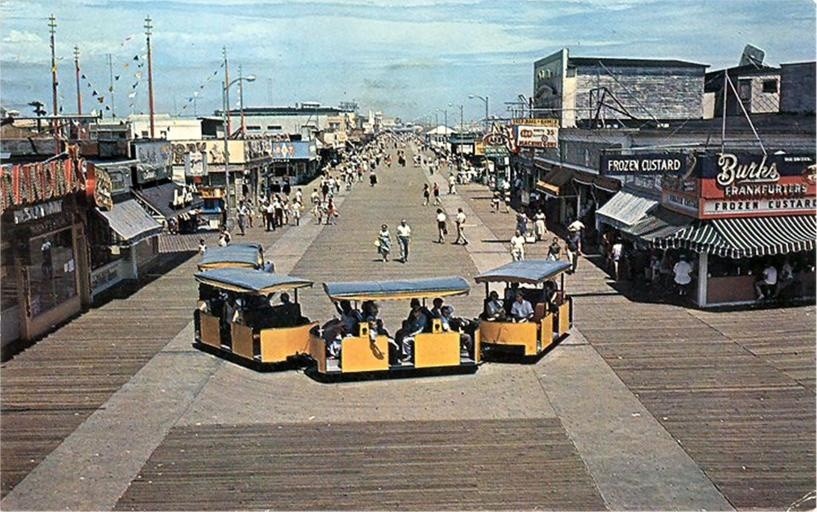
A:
[311,131,414,226]
[323,298,475,361]
[236,175,302,236]
[487,278,559,323]
[210,287,291,322]
[510,207,581,275]
[755,251,815,301]
[488,174,521,213]
[609,237,691,296]
[40,235,54,279]
[436,208,468,245]
[378,219,411,263]
[412,138,477,206]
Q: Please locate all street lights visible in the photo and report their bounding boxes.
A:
[422,101,464,174]
[467,93,488,184]
[220,75,257,230]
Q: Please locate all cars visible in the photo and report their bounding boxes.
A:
[198,195,227,228]
